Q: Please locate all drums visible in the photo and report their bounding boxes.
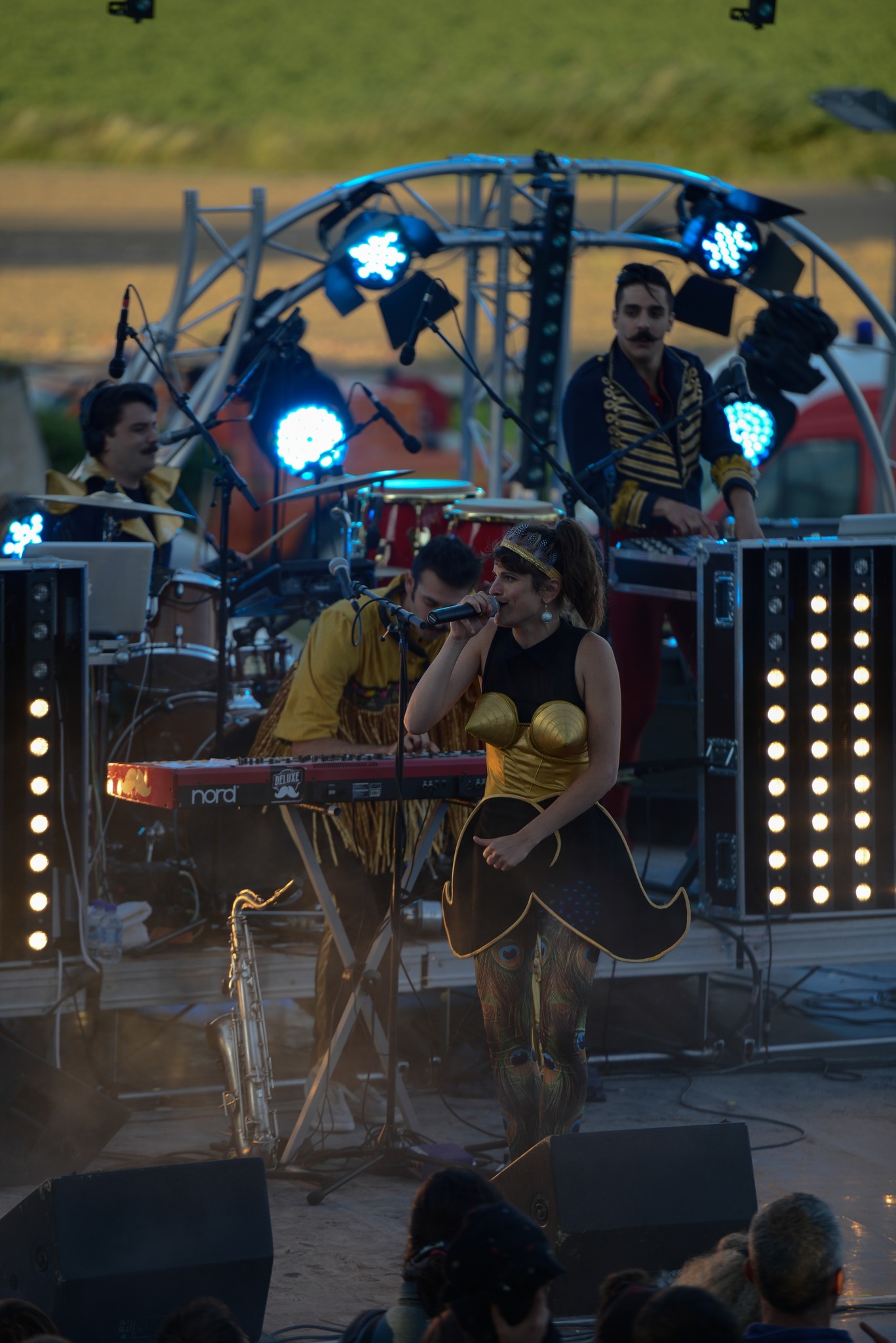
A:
[112,567,233,696]
[358,477,486,591]
[105,689,308,926]
[446,494,565,603]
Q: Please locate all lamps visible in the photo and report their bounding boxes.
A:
[721,378,797,470]
[688,194,766,284]
[249,367,355,482]
[344,209,415,294]
[2,512,54,565]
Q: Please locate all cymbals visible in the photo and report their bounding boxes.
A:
[23,489,201,522]
[267,462,414,507]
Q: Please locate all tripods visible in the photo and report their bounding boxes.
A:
[122,326,455,1206]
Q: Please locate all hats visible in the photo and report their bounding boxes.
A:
[403,1166,559,1308]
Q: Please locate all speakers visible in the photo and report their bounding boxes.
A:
[490,1122,759,1320]
[0,1156,274,1343]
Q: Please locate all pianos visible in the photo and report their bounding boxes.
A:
[101,748,490,1196]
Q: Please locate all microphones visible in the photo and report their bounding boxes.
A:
[399,280,435,366]
[728,356,752,402]
[158,421,220,445]
[363,388,422,454]
[427,594,501,625]
[329,557,359,612]
[109,288,130,378]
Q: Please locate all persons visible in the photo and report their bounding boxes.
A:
[563,263,767,856]
[250,534,484,1135]
[47,382,302,946]
[403,519,692,1163]
[0,1169,892,1343]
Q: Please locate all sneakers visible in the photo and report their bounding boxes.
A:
[302,1070,353,1132]
[344,1081,402,1123]
